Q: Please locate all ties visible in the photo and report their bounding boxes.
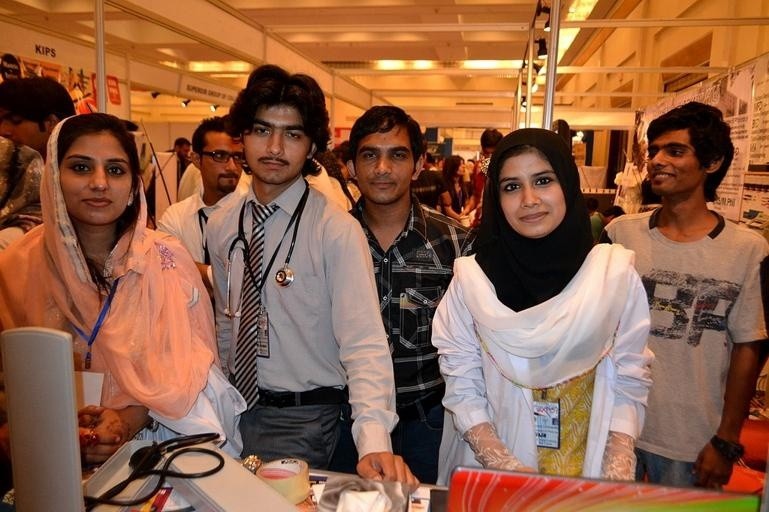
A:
[235,201,280,411]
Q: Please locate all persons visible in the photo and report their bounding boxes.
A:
[1,63,769,510]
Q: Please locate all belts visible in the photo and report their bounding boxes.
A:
[258,389,333,408]
[397,390,441,420]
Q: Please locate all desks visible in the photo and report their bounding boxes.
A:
[2,459,449,511]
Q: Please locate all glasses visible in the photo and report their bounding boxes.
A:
[201,150,244,163]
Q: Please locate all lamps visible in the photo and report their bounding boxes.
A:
[534,38,548,59]
[536,0,552,33]
[524,60,547,75]
[520,96,526,106]
[521,80,538,93]
[182,98,191,108]
[150,91,161,98]
[210,103,220,113]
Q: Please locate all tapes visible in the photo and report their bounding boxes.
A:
[256,458,311,506]
